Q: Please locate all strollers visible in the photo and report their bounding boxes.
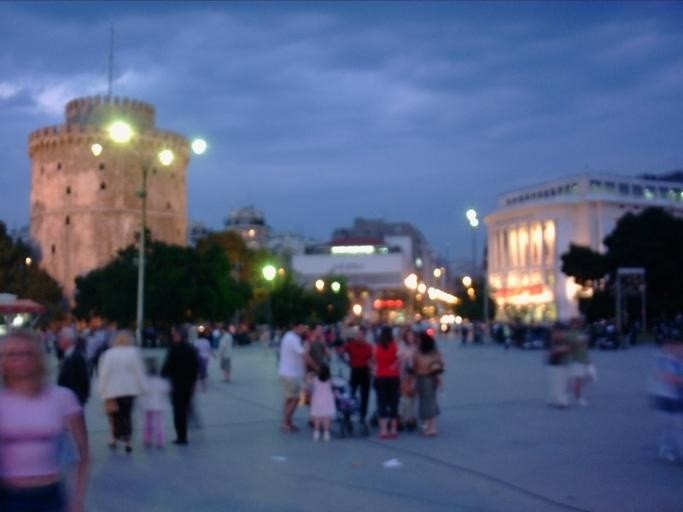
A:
[307,363,369,439]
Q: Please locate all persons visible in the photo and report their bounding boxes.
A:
[461,319,617,408]
[646,314,681,465]
[1,329,90,510]
[235,320,283,346]
[39,317,234,455]
[276,318,446,442]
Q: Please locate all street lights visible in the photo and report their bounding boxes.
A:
[465,209,478,324]
[90,119,208,346]
[315,279,341,325]
[263,264,277,342]
